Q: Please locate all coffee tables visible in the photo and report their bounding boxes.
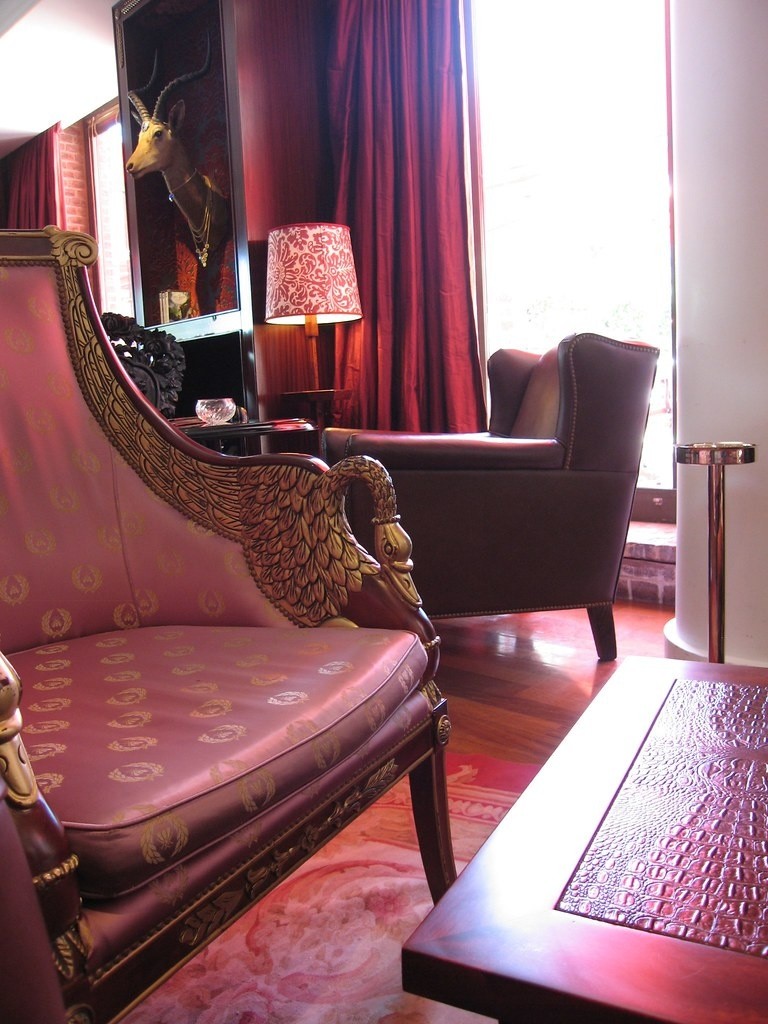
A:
[400,655,767,1024]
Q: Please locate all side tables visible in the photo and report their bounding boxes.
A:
[282,390,352,461]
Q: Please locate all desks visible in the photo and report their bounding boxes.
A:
[176,418,319,457]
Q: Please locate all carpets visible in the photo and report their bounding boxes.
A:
[118,753,546,1024]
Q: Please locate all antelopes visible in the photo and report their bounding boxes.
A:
[126,28,228,249]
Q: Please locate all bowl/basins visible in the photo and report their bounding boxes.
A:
[196,398,237,425]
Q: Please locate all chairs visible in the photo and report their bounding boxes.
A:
[321,333,661,663]
[98,312,248,456]
[0,224,458,1024]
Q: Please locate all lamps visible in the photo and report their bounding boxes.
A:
[265,224,364,390]
